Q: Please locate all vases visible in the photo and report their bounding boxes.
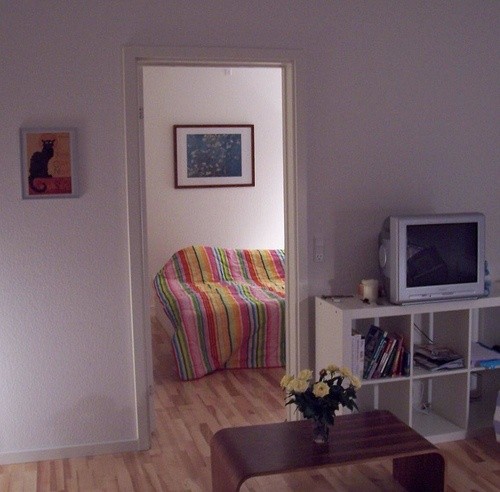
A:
[310,415,332,444]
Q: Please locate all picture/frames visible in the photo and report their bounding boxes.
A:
[173,124,255,188]
[20,128,81,199]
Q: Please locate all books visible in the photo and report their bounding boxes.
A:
[365,324,410,378]
[351,329,356,383]
[355,333,361,383]
[414,341,464,371]
[360,336,365,381]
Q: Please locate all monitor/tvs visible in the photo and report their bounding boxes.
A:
[377,212,484,305]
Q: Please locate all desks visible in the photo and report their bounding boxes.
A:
[211,410,446,492]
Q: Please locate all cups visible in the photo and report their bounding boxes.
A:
[362,279,378,302]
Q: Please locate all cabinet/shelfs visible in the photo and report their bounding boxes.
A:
[315,296,499,445]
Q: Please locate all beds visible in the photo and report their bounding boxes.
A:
[153,245,285,380]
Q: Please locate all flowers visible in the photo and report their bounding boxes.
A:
[280,365,362,441]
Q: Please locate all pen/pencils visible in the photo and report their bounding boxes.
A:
[322,294,353,298]
